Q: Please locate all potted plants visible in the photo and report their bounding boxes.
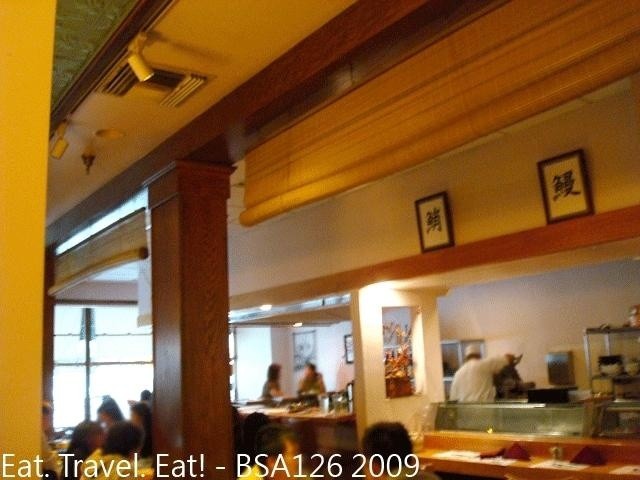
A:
[379,321,413,398]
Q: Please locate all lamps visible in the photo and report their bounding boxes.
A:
[52,118,71,159]
[126,30,155,85]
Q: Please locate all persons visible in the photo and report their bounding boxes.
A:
[491,353,525,398]
[260,364,283,400]
[296,362,326,396]
[448,343,509,405]
[230,405,441,480]
[41,389,154,480]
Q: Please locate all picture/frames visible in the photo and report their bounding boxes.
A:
[292,330,318,370]
[414,191,454,252]
[344,333,355,363]
[537,148,594,224]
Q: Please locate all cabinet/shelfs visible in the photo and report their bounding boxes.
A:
[582,326,640,402]
[438,338,484,394]
